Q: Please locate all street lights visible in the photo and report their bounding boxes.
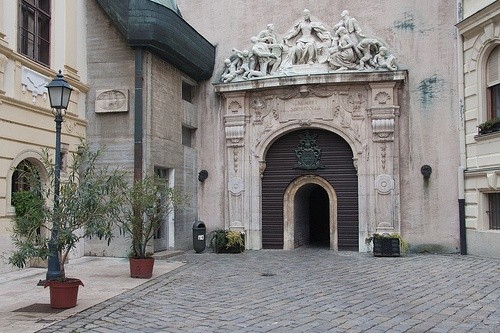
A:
[44,70,75,280]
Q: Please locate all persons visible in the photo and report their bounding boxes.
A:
[217,7,397,73]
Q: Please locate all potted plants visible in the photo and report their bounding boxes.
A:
[106,172,193,278]
[7,134,134,309]
[205,229,245,254]
[365,232,408,257]
[476,117,500,135]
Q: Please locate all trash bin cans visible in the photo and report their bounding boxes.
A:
[192,220,206,253]
